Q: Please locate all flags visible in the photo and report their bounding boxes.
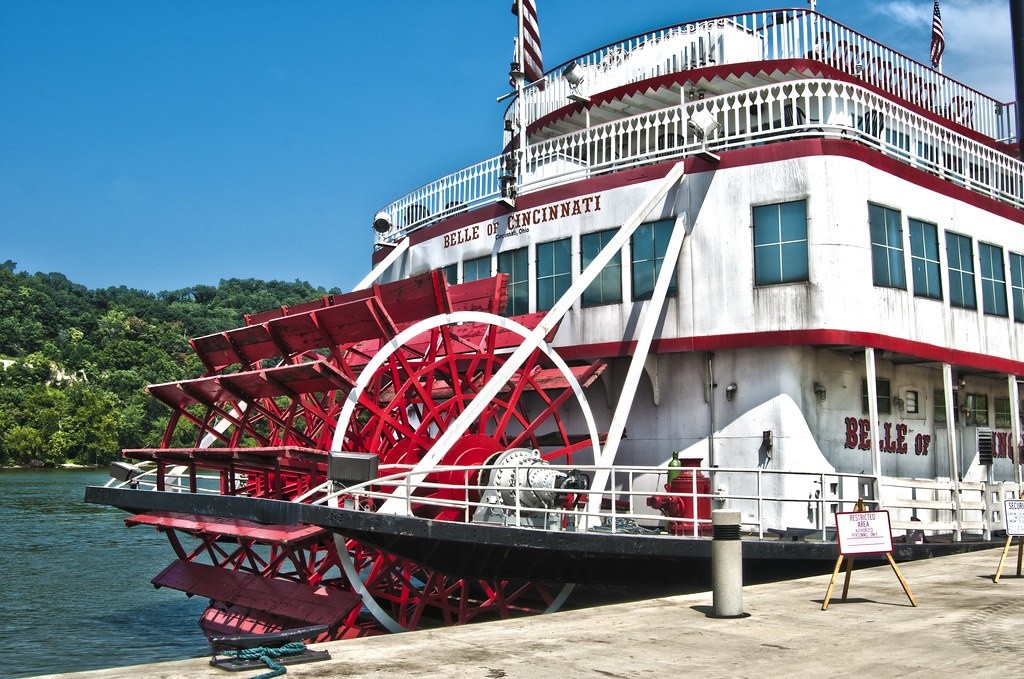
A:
[511,0,546,92]
[930,0,945,68]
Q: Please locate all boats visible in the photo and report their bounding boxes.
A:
[86,0,1024,649]
